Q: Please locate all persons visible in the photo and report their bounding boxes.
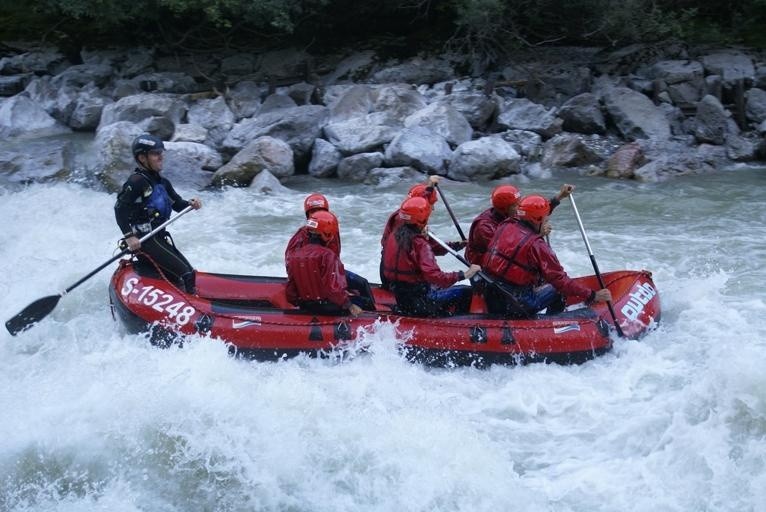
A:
[480,194,612,316]
[384,196,482,316]
[285,211,376,317]
[463,184,575,270]
[380,174,468,287]
[285,194,375,302]
[114,135,202,299]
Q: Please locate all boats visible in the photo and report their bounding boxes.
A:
[106,249,665,369]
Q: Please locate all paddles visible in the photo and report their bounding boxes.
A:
[6,200,196,336]
[426,230,530,321]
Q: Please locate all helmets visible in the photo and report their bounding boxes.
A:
[304,184,550,242]
[132,135,166,158]
